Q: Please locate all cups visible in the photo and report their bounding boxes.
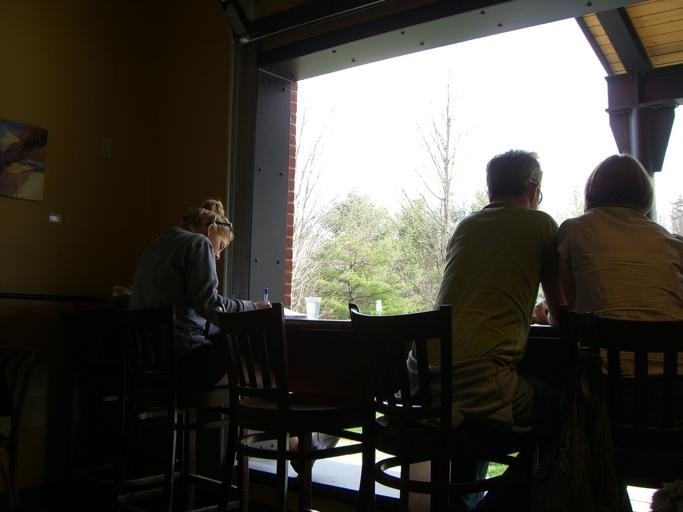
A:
[304,296,322,320]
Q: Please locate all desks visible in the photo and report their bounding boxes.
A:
[285,315,556,426]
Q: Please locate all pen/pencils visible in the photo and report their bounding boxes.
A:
[265,289,269,301]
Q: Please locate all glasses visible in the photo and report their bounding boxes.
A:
[216,222,234,230]
[527,179,543,204]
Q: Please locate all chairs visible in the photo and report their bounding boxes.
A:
[216,303,371,512]
[347,304,537,510]
[112,303,250,512]
[1,346,38,512]
[581,315,683,512]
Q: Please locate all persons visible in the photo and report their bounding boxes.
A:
[405,148,564,512]
[549,150,683,429]
[125,197,341,477]
